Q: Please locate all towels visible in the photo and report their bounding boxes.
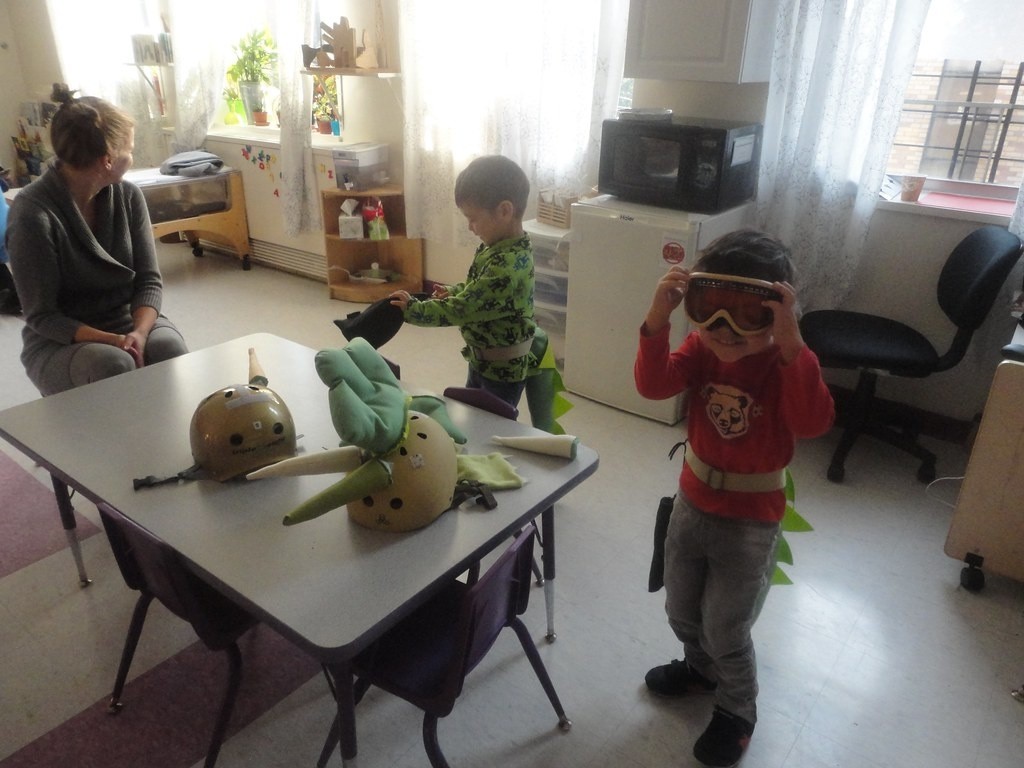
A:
[160,148,226,179]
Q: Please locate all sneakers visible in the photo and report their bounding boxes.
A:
[644,659,718,698]
[693,705,755,768]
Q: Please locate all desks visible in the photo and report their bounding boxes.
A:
[2,333,597,762]
[119,164,250,272]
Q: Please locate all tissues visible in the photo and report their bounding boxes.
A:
[338,198,364,239]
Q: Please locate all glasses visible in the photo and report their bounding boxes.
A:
[683,272,785,336]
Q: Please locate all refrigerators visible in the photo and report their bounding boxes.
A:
[563,194,747,427]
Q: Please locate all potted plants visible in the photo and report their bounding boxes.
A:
[223,24,339,137]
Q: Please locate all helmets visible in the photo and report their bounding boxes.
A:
[187,383,297,484]
[340,409,458,535]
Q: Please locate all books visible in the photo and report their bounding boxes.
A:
[11,102,59,185]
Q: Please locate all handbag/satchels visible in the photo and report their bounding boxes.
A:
[648,493,675,592]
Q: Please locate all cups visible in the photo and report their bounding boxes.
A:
[901,174,926,202]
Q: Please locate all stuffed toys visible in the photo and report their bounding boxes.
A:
[248,337,525,532]
[491,434,580,459]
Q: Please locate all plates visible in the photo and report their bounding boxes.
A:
[617,106,673,122]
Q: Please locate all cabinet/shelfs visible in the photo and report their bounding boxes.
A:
[517,216,572,358]
[621,0,773,85]
[322,180,427,302]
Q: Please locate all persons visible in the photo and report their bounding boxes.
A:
[5,82,190,396]
[389,155,574,434]
[634,227,833,768]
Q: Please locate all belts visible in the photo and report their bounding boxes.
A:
[685,441,787,492]
[468,336,535,362]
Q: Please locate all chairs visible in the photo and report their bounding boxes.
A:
[794,223,1023,483]
[312,354,571,768]
[94,502,263,767]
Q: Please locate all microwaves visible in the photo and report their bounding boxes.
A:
[598,115,763,215]
